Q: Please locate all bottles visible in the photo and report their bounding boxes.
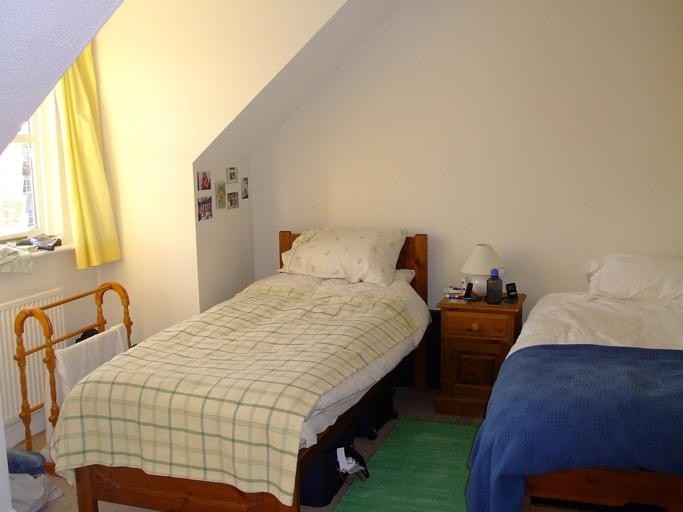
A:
[487,268,502,303]
[462,274,471,293]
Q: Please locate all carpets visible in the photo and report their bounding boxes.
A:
[333,415,479,512]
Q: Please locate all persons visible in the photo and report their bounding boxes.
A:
[202,172,210,189]
[199,199,212,220]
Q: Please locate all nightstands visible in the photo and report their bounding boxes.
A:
[434,290,525,420]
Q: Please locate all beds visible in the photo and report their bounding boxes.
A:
[61,231,428,510]
[474,291,680,511]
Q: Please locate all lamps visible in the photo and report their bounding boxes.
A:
[459,241,507,299]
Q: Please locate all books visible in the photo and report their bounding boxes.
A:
[444,287,464,299]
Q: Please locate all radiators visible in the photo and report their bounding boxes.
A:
[1,287,66,429]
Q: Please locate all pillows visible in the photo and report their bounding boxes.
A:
[279,222,409,288]
[581,253,681,302]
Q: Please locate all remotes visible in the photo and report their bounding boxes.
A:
[464,283,473,298]
